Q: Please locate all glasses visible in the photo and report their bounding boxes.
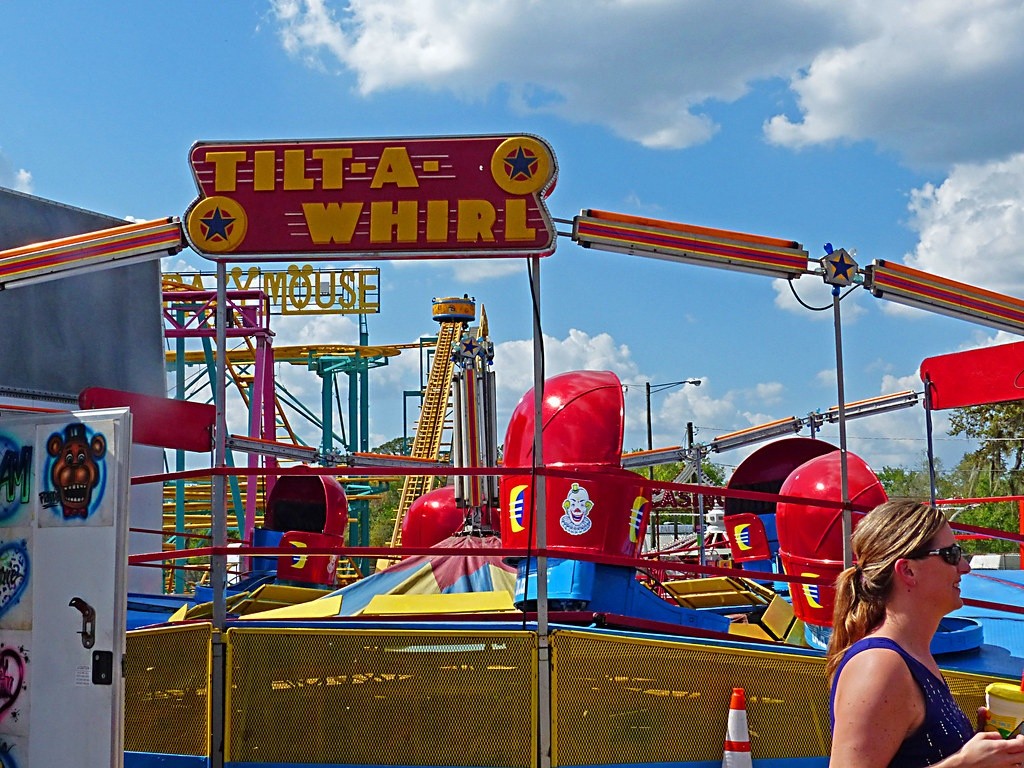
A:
[902,544,962,566]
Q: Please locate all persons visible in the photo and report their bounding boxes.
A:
[824,500,1024,768]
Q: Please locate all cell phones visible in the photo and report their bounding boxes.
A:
[1006,721,1024,740]
